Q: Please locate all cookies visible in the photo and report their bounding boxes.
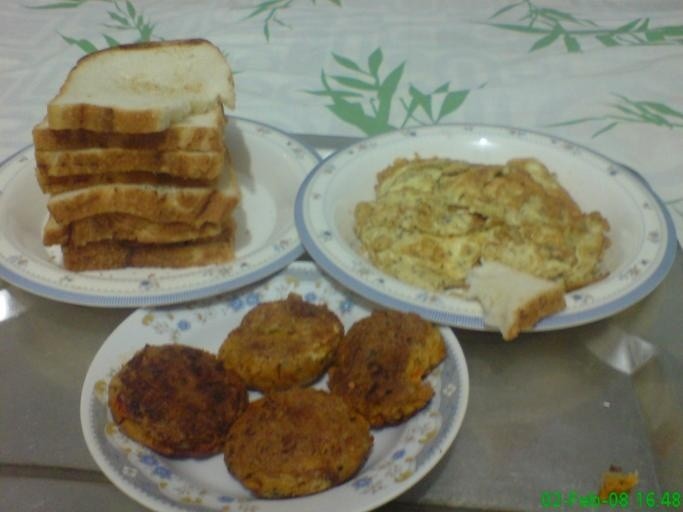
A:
[222,387,374,500]
[327,308,448,427]
[108,342,249,460]
[218,293,344,394]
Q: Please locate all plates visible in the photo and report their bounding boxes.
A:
[295,121,678,336]
[0,104,330,304]
[76,255,473,512]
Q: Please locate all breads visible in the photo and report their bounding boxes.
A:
[467,261,565,340]
[32,39,238,271]
